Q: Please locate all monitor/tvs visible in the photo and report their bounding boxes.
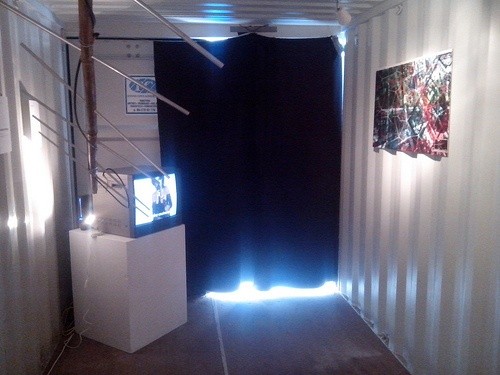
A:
[92,165,182,238]
